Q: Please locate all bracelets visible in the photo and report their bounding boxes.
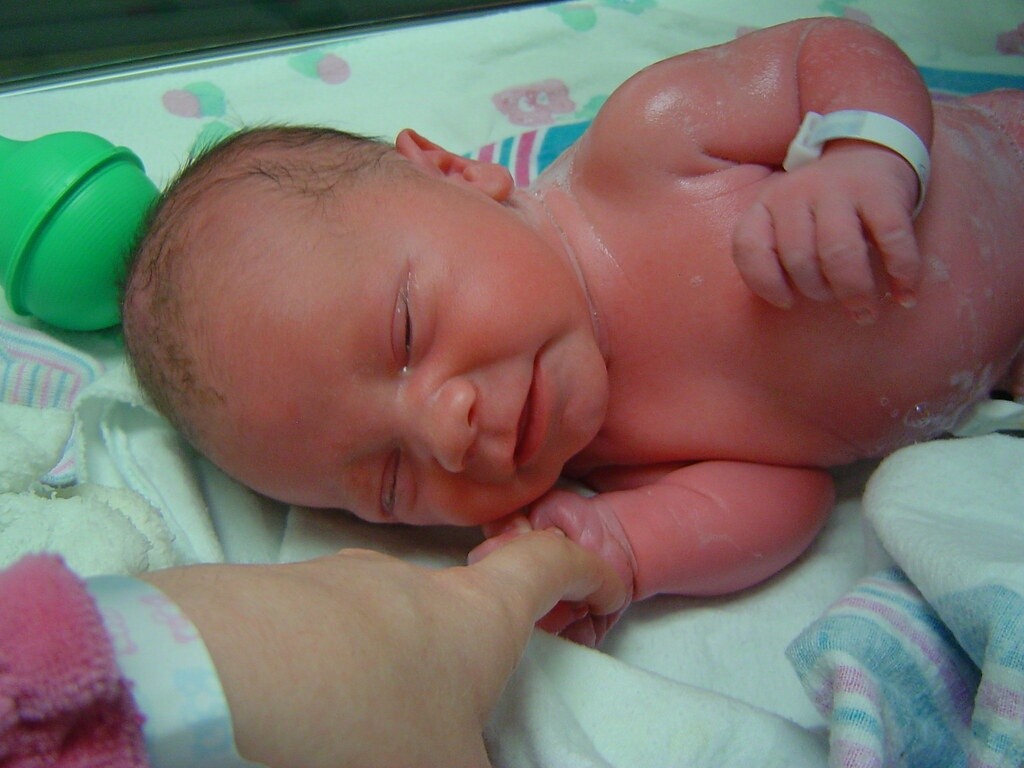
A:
[779,109,929,222]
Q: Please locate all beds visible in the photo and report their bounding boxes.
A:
[0,0,1024,768]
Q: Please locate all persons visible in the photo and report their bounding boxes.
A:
[124,19,1024,650]
[0,525,635,768]
